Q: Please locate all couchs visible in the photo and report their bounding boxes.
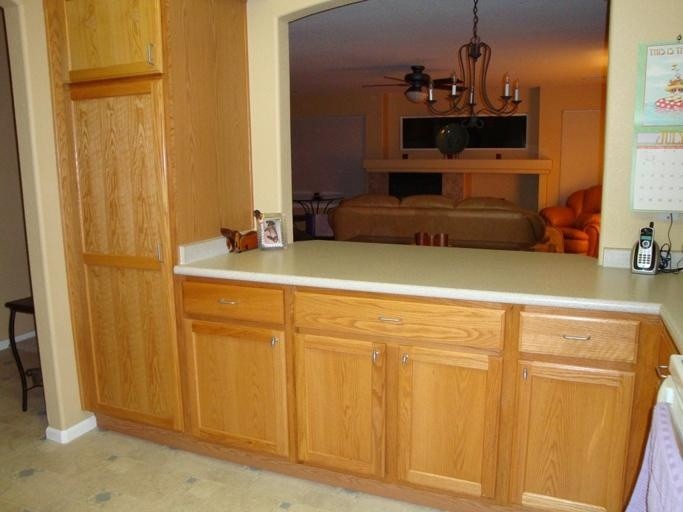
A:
[541,186,604,257]
[330,195,545,249]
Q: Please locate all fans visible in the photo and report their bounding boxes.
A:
[360,65,471,91]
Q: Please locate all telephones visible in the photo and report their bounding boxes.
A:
[631,222,660,275]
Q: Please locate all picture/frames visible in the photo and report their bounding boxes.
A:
[252,208,294,255]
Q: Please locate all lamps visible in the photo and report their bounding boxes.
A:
[404,81,428,103]
[426,2,522,114]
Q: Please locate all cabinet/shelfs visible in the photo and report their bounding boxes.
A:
[171,279,289,460]
[288,293,391,479]
[509,306,683,509]
[390,298,510,495]
[42,0,256,429]
